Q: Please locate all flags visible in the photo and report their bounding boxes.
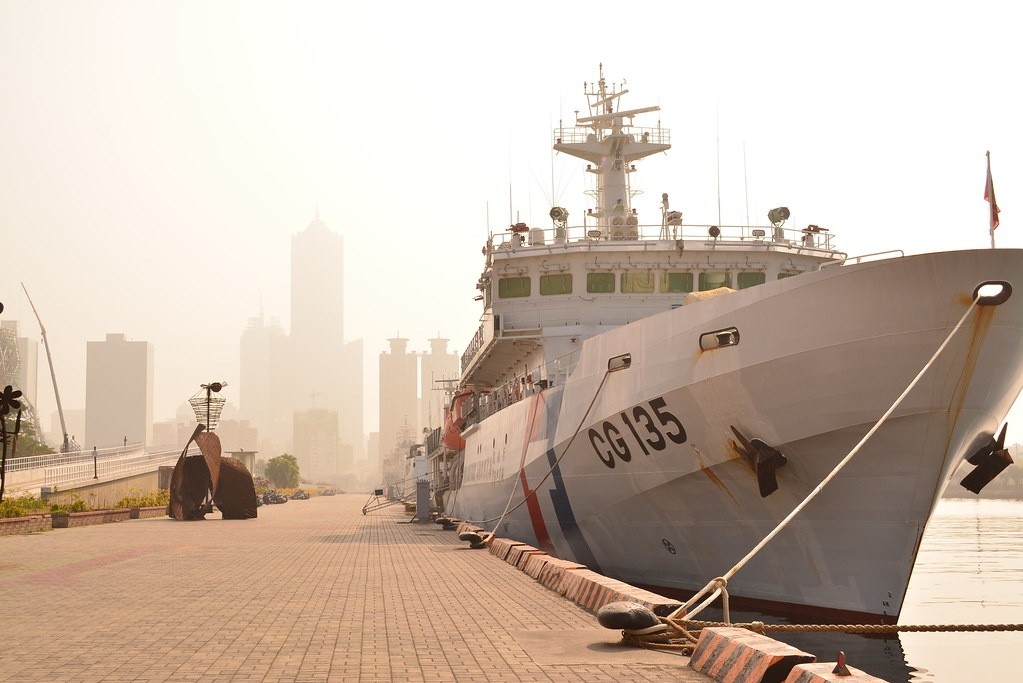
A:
[984,168,1000,235]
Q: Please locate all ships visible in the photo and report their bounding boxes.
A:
[400,63,1023,635]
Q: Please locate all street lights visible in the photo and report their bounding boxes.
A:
[201,382,224,434]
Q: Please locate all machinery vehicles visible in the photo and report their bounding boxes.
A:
[21,282,79,454]
